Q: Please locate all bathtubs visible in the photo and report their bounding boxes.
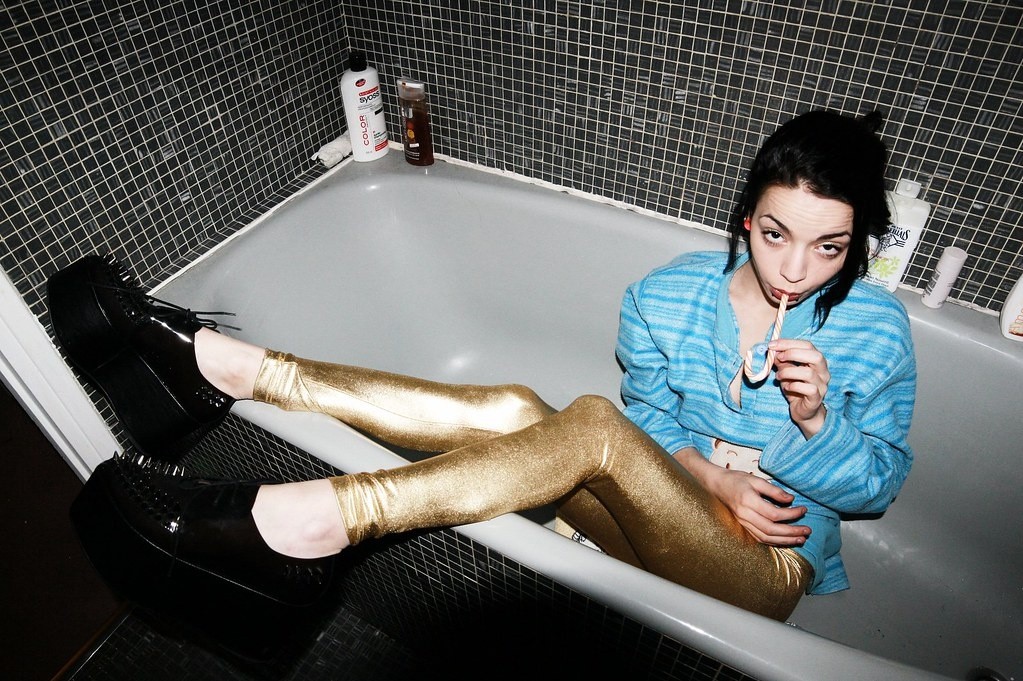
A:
[89,132,1023,681]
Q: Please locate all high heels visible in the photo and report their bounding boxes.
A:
[76,452,355,677]
[45,255,237,465]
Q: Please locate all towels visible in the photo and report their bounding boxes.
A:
[311,130,355,170]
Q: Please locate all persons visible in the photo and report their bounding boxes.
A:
[41,108,920,670]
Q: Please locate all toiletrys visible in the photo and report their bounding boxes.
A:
[339,50,391,164]
[863,177,932,300]
[997,269,1023,346]
[921,246,969,309]
[396,76,436,167]
